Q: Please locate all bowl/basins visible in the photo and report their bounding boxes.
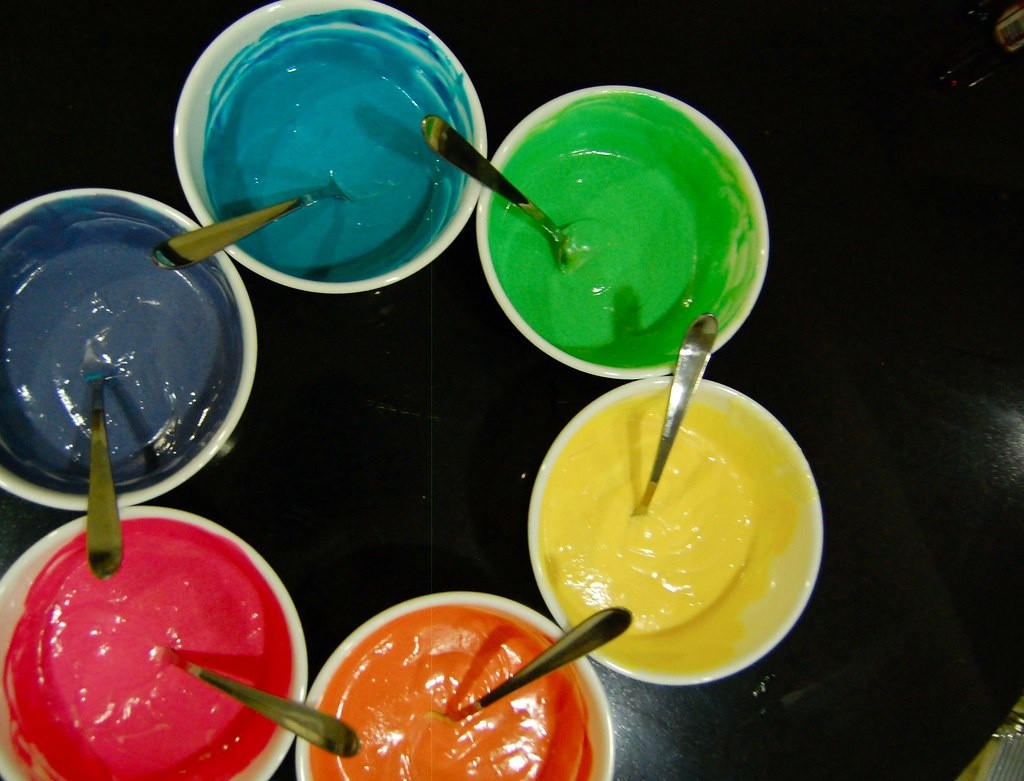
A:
[475,86,771,380]
[174,0,488,294]
[0,188,259,512]
[528,376,824,686]
[296,589,615,781]
[0,506,308,781]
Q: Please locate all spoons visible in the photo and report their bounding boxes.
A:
[153,168,367,276]
[79,332,131,578]
[422,117,601,270]
